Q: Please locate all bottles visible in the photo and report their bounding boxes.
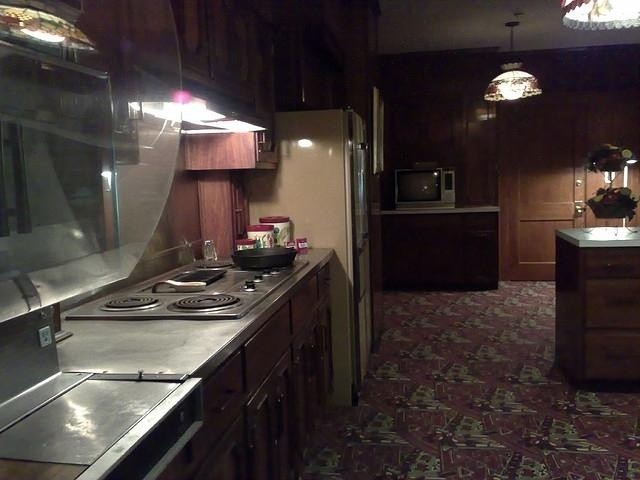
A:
[257,214,293,248]
[244,222,275,251]
[204,240,218,261]
[235,239,258,252]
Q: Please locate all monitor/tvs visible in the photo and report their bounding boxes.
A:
[393,167,456,211]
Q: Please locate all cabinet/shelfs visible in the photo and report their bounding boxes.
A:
[241,275,320,480]
[554,237,639,383]
[197,170,249,257]
[122,1,271,134]
[382,205,501,291]
[184,16,279,170]
[182,350,247,480]
[318,264,335,399]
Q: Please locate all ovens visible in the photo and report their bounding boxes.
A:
[255,123,280,163]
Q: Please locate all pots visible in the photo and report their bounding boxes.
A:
[229,246,299,271]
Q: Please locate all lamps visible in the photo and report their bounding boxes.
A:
[558,0,639,32]
[483,21,544,102]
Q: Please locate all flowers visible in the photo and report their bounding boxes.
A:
[583,143,639,221]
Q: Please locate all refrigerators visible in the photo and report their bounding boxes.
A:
[245,106,378,414]
